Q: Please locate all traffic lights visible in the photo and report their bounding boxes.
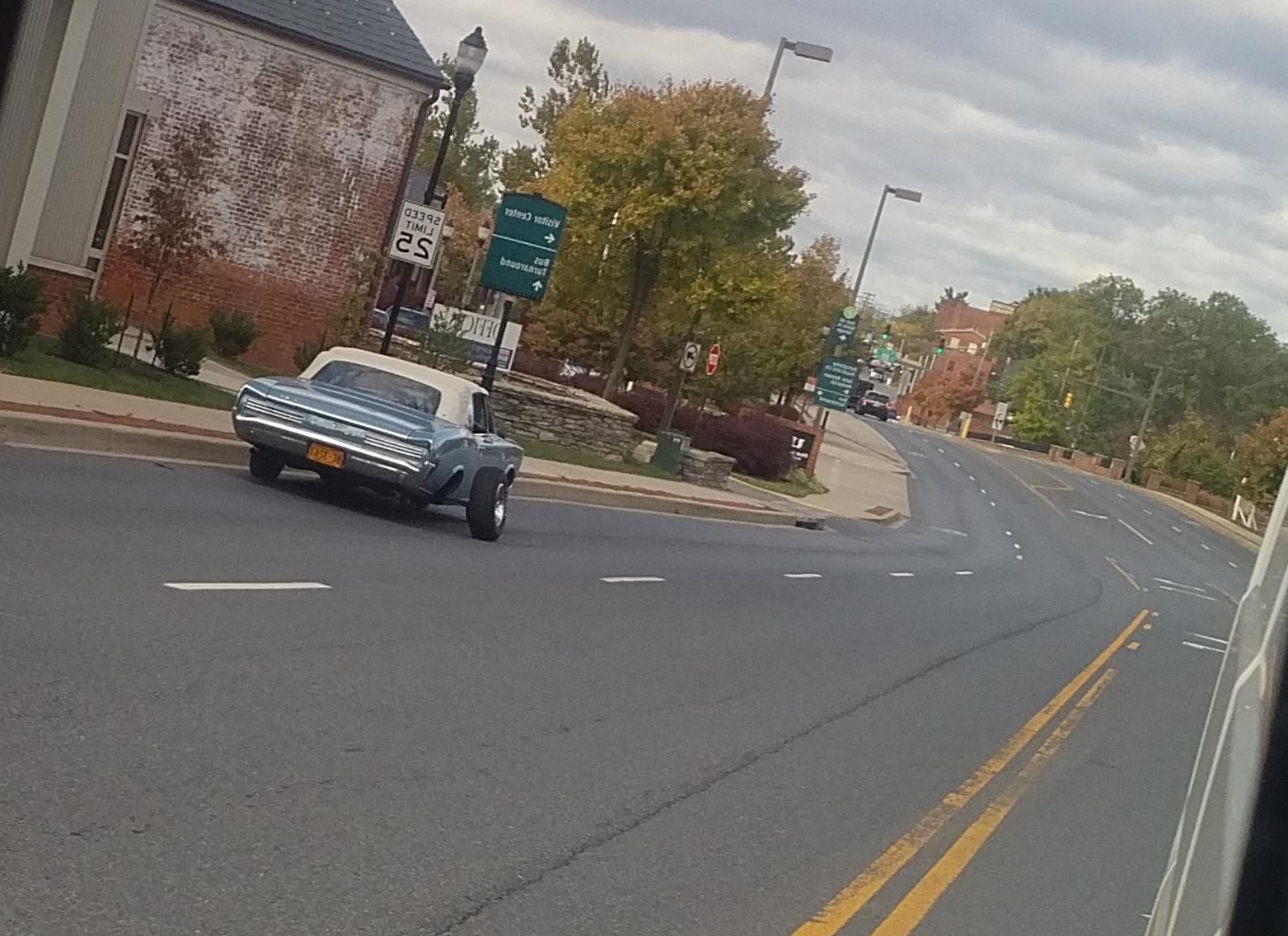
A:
[881,324,891,340]
[936,338,945,354]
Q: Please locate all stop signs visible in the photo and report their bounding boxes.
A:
[707,344,724,376]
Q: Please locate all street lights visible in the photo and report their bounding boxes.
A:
[377,23,490,356]
[811,182,921,425]
[655,36,840,434]
[1122,363,1163,483]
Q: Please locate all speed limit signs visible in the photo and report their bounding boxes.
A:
[388,200,445,270]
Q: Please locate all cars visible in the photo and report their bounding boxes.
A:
[230,345,525,543]
[852,356,902,422]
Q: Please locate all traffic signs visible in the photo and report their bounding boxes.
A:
[478,192,568,302]
